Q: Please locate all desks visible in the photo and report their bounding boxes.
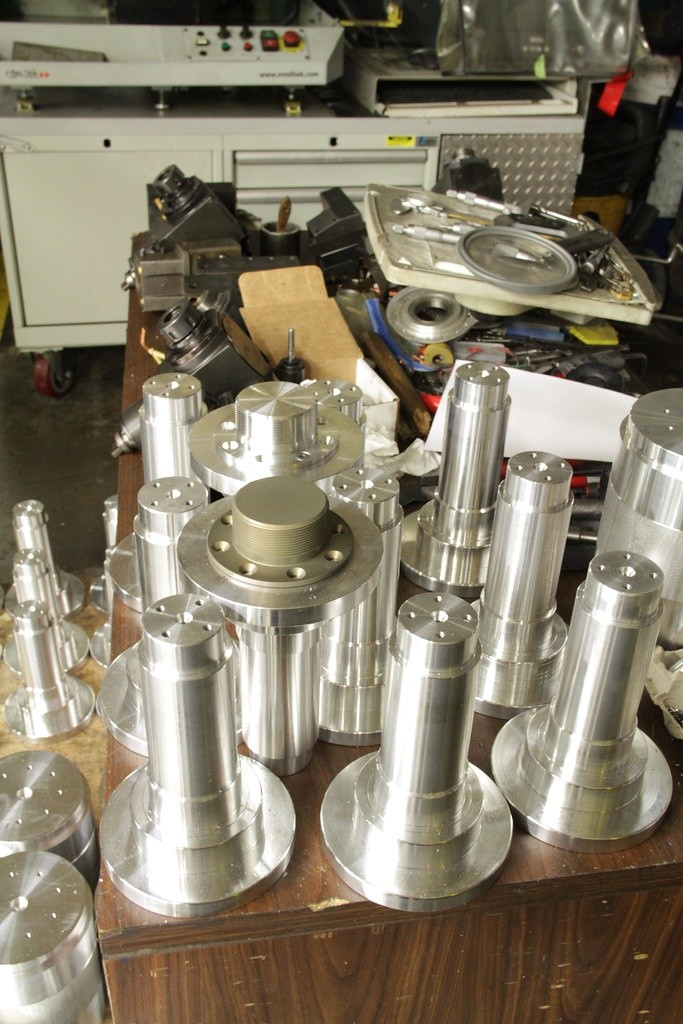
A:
[91,230,683,1024]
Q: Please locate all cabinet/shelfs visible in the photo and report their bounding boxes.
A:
[0,86,447,398]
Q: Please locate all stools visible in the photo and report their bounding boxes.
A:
[571,195,629,237]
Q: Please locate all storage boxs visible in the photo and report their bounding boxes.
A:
[236,262,403,441]
